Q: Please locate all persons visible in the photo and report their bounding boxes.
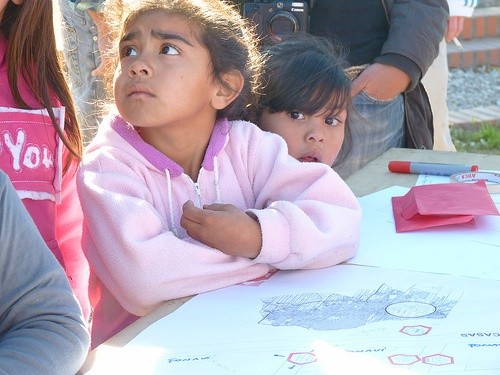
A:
[249,28,356,169]
[74,1,364,349]
[215,0,450,177]
[420,0,478,151]
[0,169,90,375]
[0,0,92,325]
[57,0,115,144]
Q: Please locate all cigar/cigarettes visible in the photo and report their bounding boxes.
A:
[453,37,463,48]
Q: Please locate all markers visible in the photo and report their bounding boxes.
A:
[388,161,477,176]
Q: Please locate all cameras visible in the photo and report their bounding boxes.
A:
[243,2,310,44]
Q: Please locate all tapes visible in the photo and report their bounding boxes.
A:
[450,170,500,192]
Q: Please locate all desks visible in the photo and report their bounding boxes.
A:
[79,146,500,375]
